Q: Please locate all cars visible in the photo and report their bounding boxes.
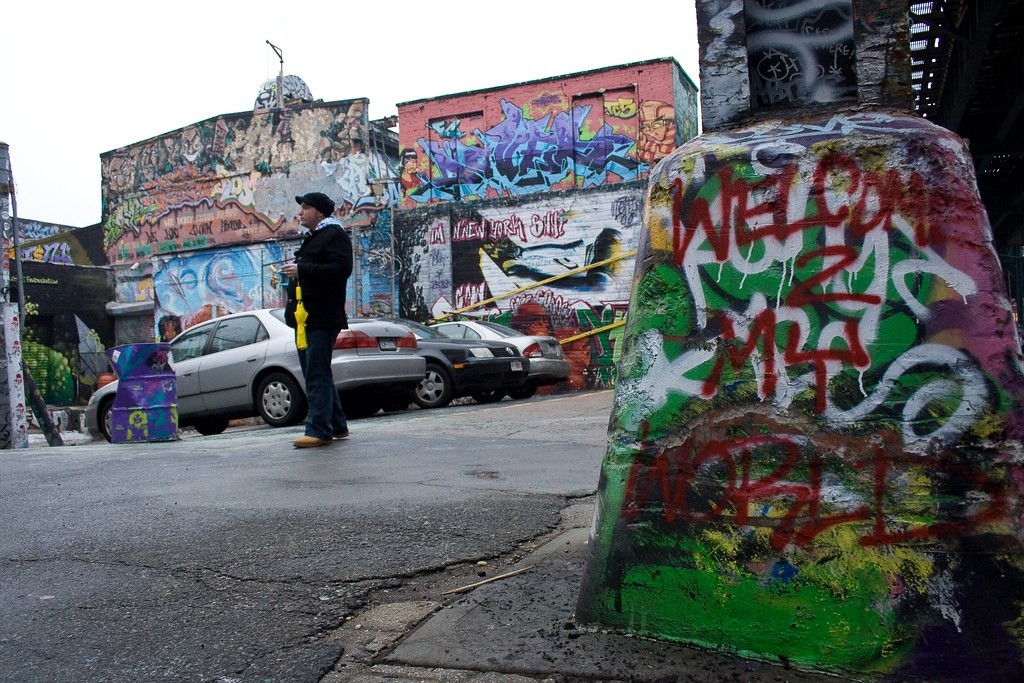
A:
[85,306,428,444]
[428,319,572,402]
[345,317,531,408]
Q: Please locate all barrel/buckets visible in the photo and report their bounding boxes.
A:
[105,342,179,443]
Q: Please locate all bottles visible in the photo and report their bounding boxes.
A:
[98,373,113,389]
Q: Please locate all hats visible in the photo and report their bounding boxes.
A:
[295,192,336,217]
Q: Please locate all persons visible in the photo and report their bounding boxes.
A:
[279,192,353,448]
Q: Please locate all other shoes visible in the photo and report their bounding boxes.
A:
[293,435,332,447]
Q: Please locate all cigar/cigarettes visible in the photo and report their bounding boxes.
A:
[277,269,282,273]
